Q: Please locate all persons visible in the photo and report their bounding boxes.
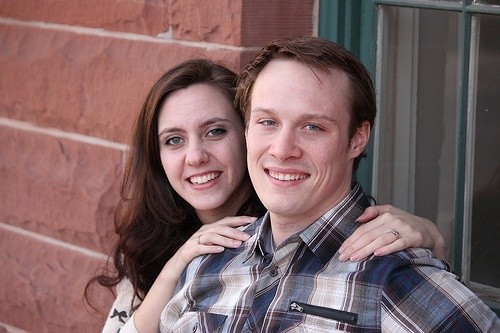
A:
[84,58,444,333]
[158,33,500,333]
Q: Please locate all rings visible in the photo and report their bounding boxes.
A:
[197,233,203,244]
[387,229,399,240]
